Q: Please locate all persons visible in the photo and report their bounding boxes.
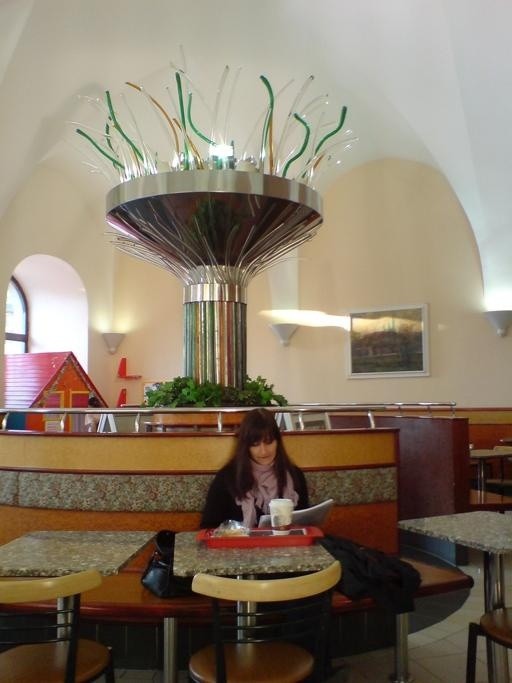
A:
[197,408,339,681]
[83,396,102,434]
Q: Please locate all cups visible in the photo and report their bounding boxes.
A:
[268,497,294,534]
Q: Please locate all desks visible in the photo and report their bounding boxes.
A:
[0,525,156,681]
[172,529,335,640]
[397,510,512,682]
[470,445,511,491]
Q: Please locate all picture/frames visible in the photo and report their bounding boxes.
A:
[344,301,430,380]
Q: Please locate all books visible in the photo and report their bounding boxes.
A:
[253,496,337,535]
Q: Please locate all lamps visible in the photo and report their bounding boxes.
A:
[102,332,126,355]
[481,309,512,336]
[270,322,298,348]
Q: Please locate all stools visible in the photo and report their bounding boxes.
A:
[468,606,512,682]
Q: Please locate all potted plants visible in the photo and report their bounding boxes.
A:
[140,374,291,431]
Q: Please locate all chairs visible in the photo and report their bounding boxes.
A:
[483,444,512,487]
[0,568,116,681]
[186,558,345,682]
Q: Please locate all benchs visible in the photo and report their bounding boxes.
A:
[0,548,474,681]
[470,486,512,511]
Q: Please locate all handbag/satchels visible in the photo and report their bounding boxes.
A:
[141,529,194,599]
